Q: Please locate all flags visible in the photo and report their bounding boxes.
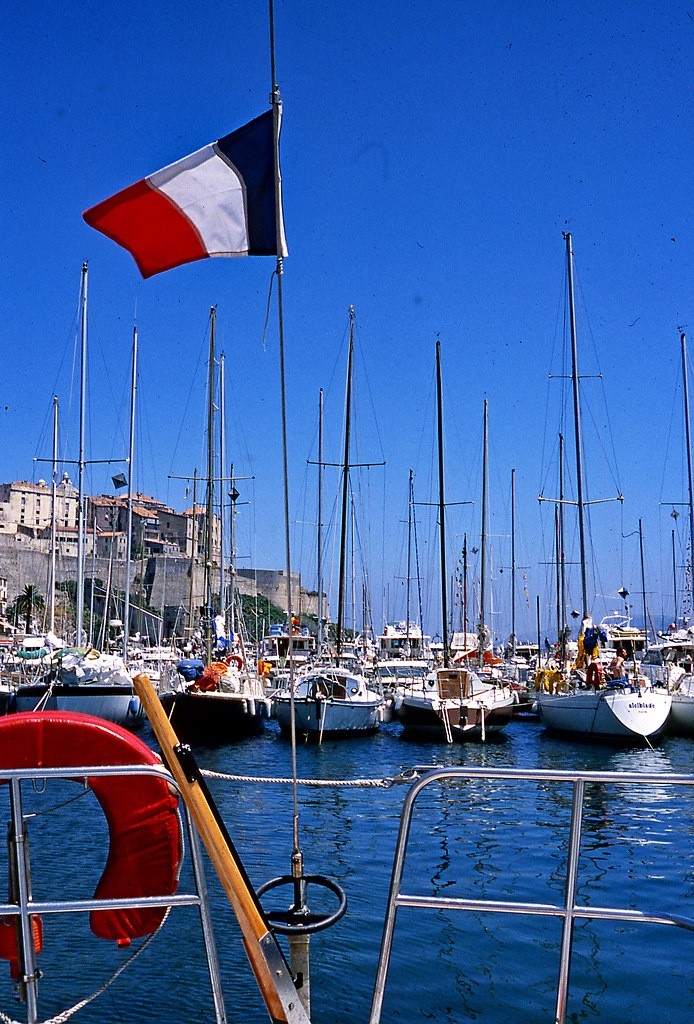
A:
[80,93,288,278]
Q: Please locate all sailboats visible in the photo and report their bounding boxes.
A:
[0,220,694,753]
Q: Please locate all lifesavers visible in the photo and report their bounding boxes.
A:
[259,660,264,674]
[534,669,561,695]
[226,656,242,671]
[0,711,181,960]
[587,664,602,686]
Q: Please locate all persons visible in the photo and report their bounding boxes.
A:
[608,646,628,681]
